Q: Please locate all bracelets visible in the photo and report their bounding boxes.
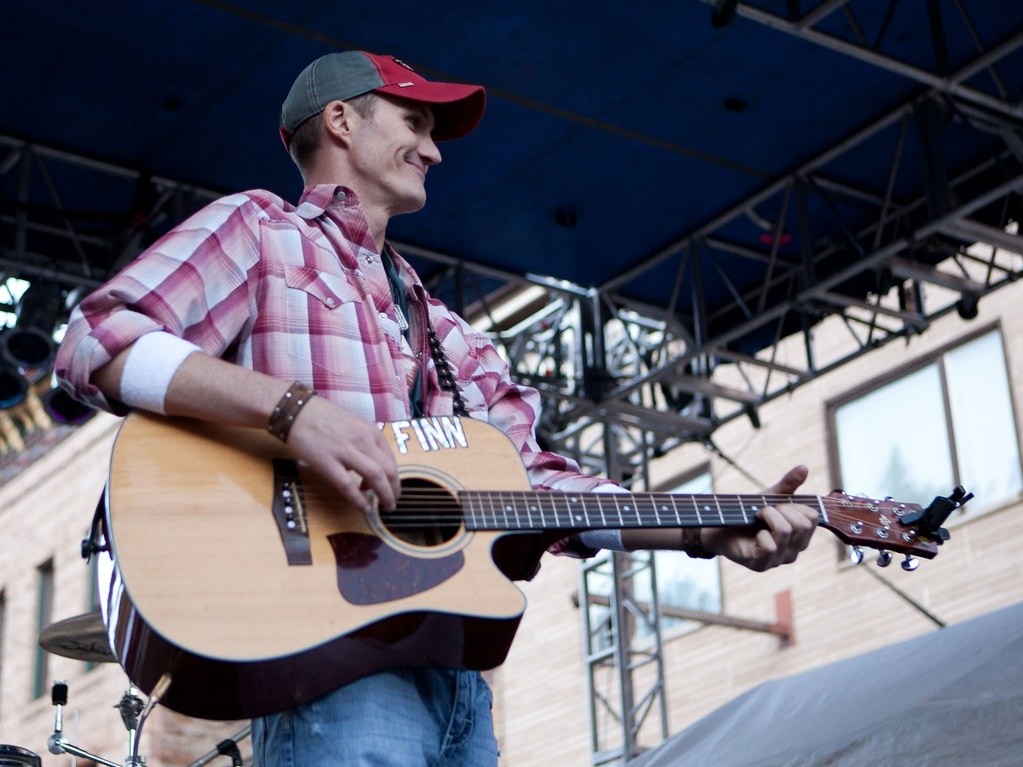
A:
[683,526,716,559]
[267,379,319,443]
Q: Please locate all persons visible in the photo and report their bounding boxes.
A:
[56,52,819,767]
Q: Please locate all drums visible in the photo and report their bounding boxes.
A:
[0,744,42,767]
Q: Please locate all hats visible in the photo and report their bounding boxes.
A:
[279,51,487,156]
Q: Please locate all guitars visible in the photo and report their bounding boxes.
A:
[87,403,983,725]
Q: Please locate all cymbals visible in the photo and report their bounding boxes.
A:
[36,609,120,664]
